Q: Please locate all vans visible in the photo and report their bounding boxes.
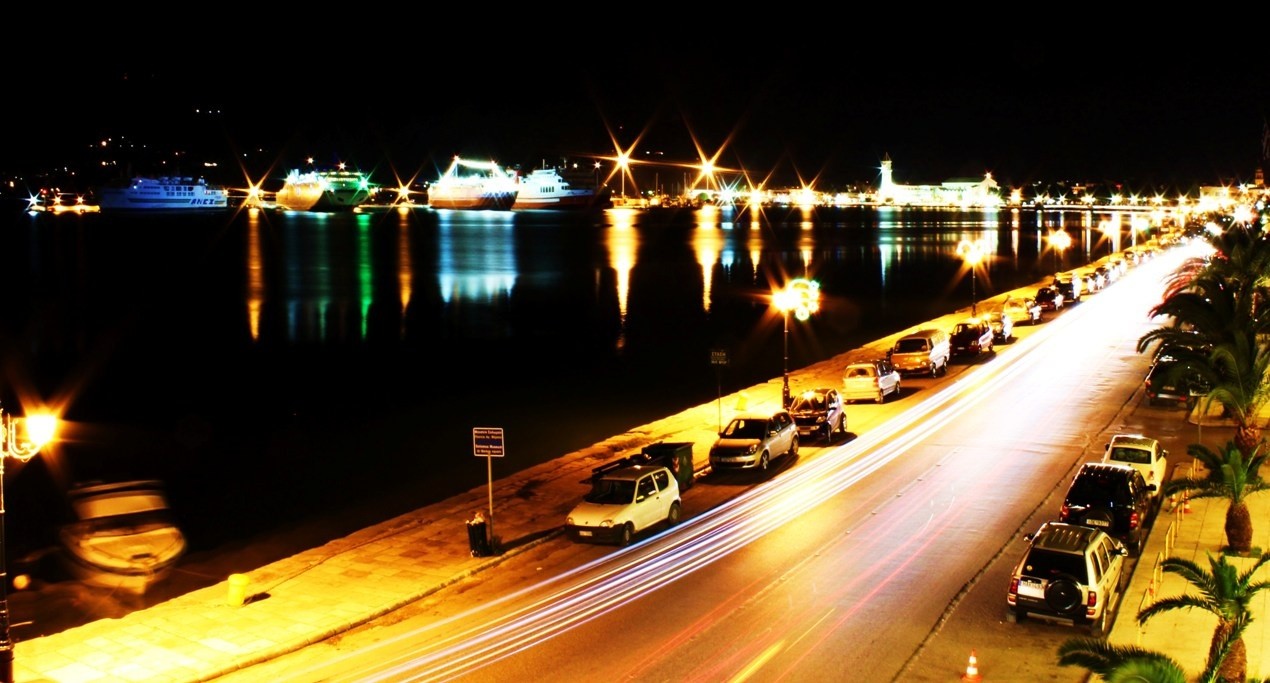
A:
[889,329,950,380]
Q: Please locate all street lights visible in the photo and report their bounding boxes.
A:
[966,248,982,317]
[773,290,798,411]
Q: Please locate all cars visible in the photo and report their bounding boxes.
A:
[1143,330,1216,414]
[1003,296,1044,326]
[1034,234,1179,312]
[981,311,1013,345]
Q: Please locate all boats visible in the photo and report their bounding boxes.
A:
[427,154,593,210]
[29,188,100,215]
[66,475,172,523]
[99,177,228,211]
[275,169,371,212]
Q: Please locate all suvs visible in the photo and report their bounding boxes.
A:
[563,463,683,550]
[843,359,901,405]
[709,406,800,477]
[948,318,994,359]
[788,388,847,446]
[1057,462,1157,558]
[1101,434,1170,502]
[1007,518,1129,638]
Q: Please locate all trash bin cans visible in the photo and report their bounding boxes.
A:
[591,442,695,491]
[466,522,488,556]
